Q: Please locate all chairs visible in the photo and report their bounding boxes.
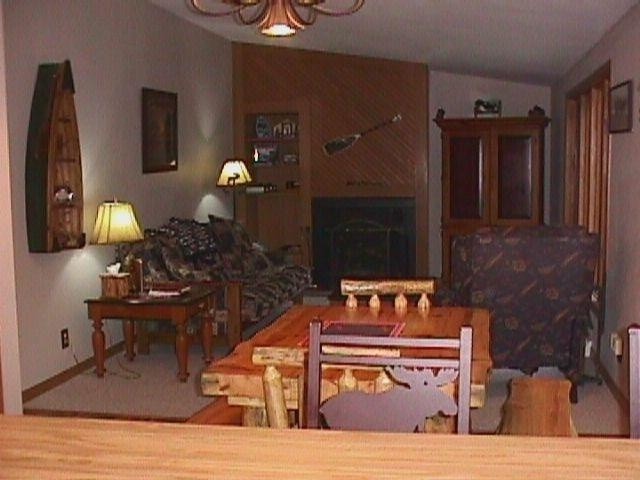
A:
[341,278,435,310]
[307,323,473,435]
[251,346,400,430]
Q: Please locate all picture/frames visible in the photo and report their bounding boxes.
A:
[143,87,179,175]
[609,80,633,135]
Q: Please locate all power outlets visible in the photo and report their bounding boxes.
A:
[609,333,618,350]
[613,337,622,356]
[61,328,69,349]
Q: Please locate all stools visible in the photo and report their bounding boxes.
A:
[495,379,577,438]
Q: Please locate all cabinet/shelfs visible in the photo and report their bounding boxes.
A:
[432,105,552,281]
[234,98,310,268]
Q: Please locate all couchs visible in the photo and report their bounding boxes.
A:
[433,225,601,404]
[114,215,312,356]
[182,396,243,424]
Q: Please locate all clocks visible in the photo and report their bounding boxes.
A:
[25,58,86,254]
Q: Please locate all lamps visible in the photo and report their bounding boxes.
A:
[216,159,252,221]
[89,198,145,274]
[192,1,364,36]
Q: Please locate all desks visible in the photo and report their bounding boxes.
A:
[83,288,216,382]
[200,304,490,407]
[0,415,640,480]
